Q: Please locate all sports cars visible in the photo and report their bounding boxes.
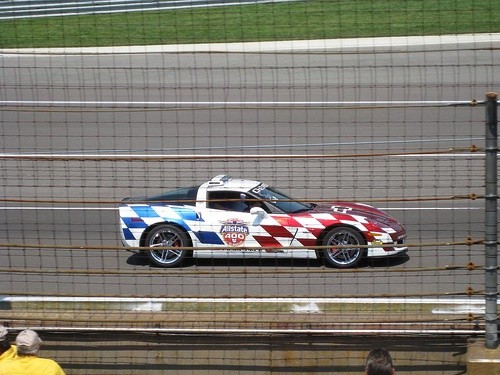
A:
[119,174,409,269]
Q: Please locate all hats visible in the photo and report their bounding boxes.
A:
[15,330,42,351]
[0,325,9,341]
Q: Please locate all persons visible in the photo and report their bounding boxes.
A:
[0,325,66,375]
[228,191,262,213]
